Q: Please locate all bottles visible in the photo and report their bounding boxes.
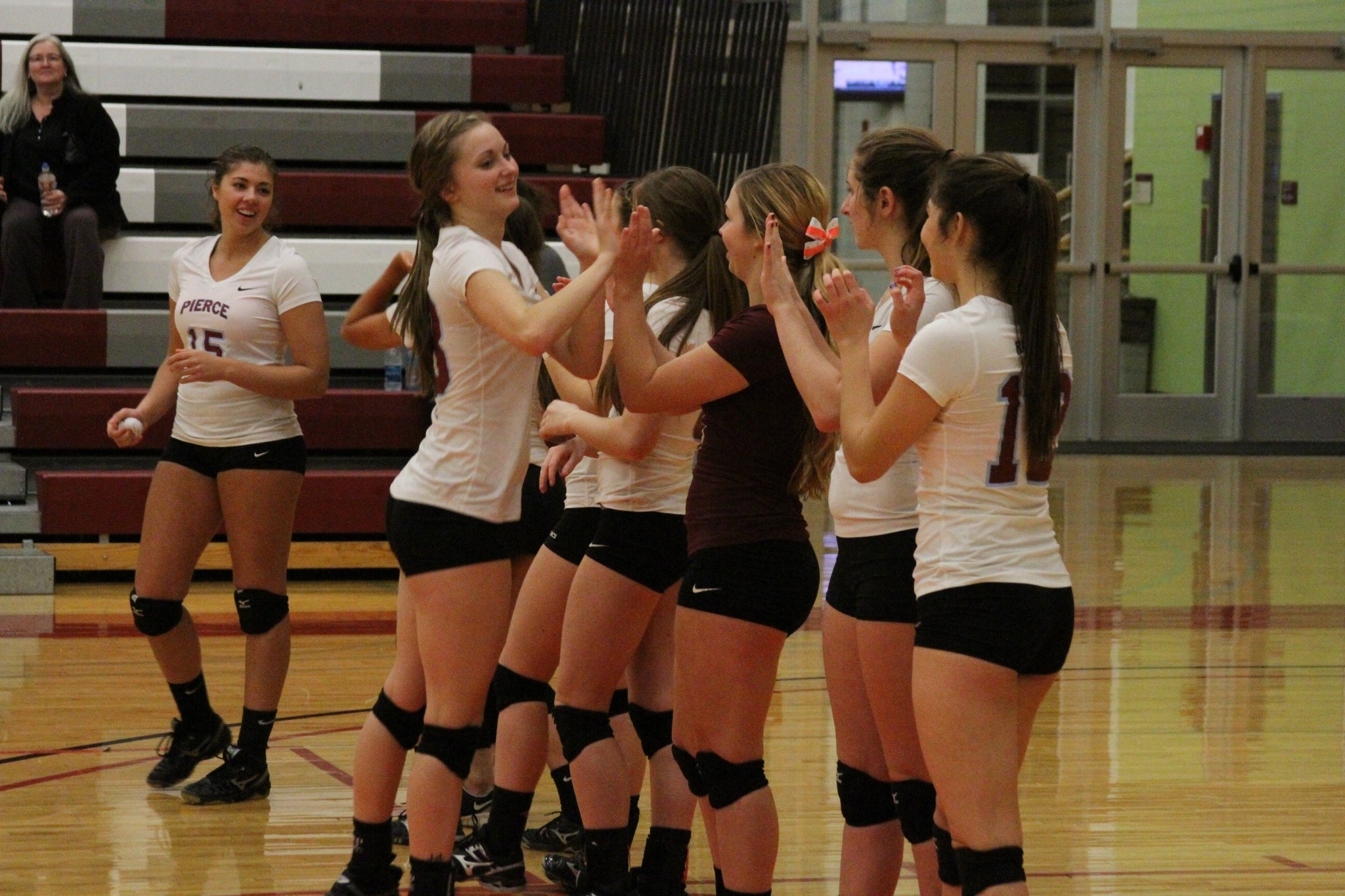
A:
[38,162,59,218]
[404,349,421,391]
[383,346,403,392]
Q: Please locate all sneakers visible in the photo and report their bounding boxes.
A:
[391,800,466,846]
[325,864,404,896]
[181,743,271,805]
[148,712,232,789]
[520,810,584,854]
[461,786,493,826]
[450,814,527,892]
[541,851,587,892]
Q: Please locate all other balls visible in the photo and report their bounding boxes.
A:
[118,417,143,437]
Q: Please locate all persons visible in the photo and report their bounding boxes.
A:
[106,147,333,805]
[331,108,624,895]
[0,33,131,310]
[812,152,1076,896]
[341,127,961,896]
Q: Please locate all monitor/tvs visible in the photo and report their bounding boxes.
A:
[835,60,906,101]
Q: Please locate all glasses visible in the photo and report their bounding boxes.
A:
[28,53,63,61]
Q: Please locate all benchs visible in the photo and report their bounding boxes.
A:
[0,1,625,543]
